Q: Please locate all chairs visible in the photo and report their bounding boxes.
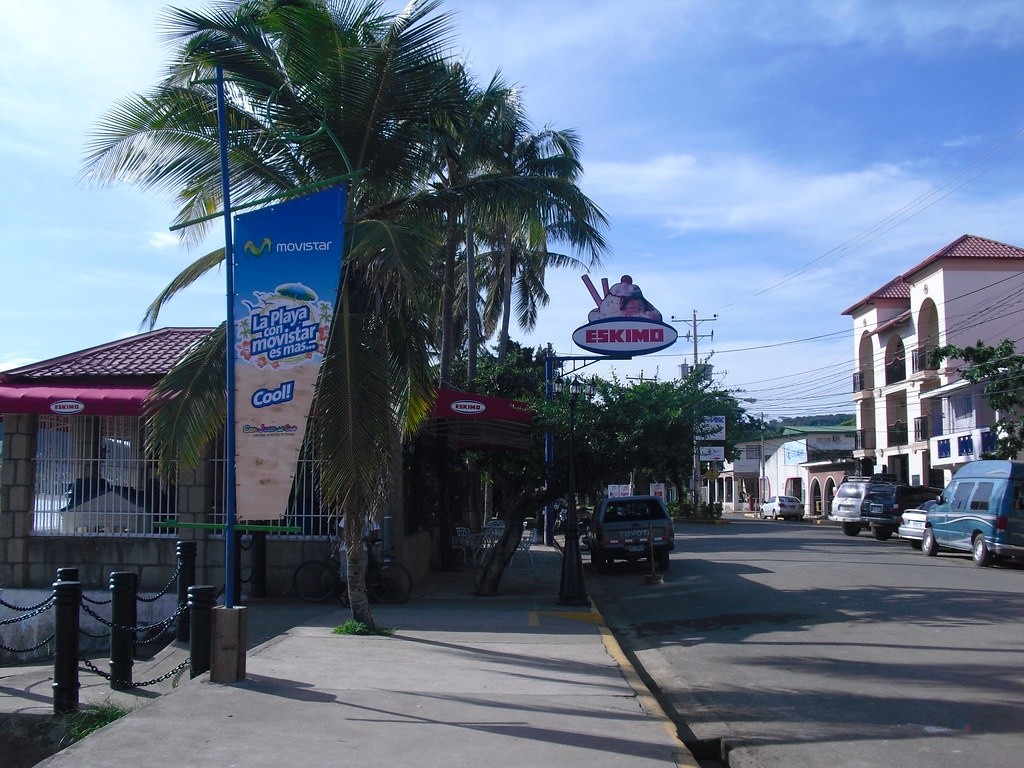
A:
[451,518,537,569]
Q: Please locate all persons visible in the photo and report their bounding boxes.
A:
[702,501,713,518]
[717,498,723,513]
[739,488,746,502]
[437,499,466,572]
[578,504,593,529]
[540,514,545,545]
[64,475,87,498]
[339,505,381,608]
[60,461,110,512]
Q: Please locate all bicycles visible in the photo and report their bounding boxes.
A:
[292,529,413,608]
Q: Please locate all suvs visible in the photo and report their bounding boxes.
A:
[859,483,945,549]
[830,474,905,534]
[587,494,675,574]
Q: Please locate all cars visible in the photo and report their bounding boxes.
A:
[760,495,805,521]
[899,500,936,549]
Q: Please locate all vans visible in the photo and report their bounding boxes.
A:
[922,460,1024,567]
